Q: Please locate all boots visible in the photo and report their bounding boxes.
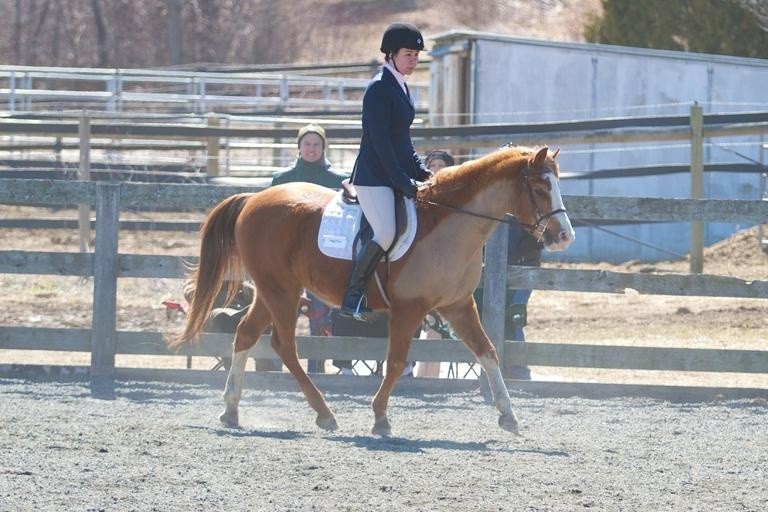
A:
[340,240,387,318]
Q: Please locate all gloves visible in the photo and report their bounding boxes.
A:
[400,180,418,199]
[509,303,527,330]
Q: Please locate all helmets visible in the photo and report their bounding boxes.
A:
[380,21,428,51]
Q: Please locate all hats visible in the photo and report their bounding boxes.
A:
[297,121,327,151]
[424,151,454,170]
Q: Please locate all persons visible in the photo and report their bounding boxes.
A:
[272,124,358,375]
[397,149,457,378]
[184,272,273,371]
[473,147,542,381]
[340,22,427,321]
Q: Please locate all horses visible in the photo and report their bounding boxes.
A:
[161,143,576,437]
